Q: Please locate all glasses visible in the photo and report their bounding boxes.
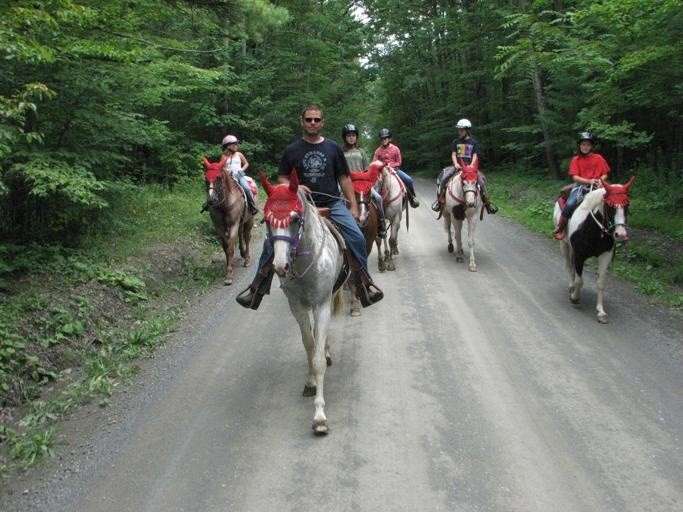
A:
[304,116,322,123]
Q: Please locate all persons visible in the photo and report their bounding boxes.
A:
[372,128,419,207]
[217,134,259,218]
[336,124,387,237]
[433,119,500,214]
[236,105,384,310]
[552,131,610,235]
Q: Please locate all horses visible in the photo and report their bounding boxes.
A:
[345,170,376,318]
[363,159,404,272]
[552,175,637,325]
[441,156,482,273]
[258,168,353,436]
[201,155,259,287]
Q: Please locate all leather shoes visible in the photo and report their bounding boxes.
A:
[238,291,262,311]
[360,288,384,308]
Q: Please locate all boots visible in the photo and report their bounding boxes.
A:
[555,216,567,240]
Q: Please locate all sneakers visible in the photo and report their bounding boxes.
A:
[410,196,419,208]
[486,203,498,215]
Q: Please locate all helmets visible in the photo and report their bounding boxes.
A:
[221,134,240,145]
[576,130,596,145]
[455,117,472,130]
[341,124,358,139]
[378,127,391,139]
